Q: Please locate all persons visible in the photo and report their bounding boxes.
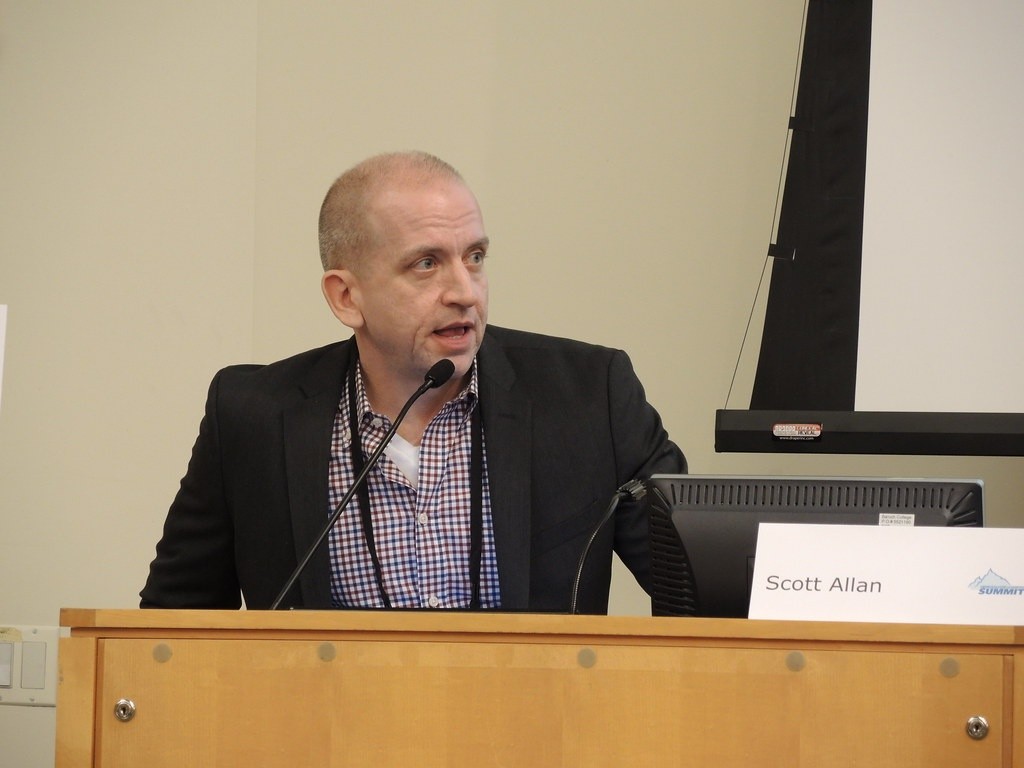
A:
[141,150,689,618]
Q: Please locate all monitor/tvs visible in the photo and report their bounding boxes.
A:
[646,475,982,619]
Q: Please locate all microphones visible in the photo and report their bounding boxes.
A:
[273,358,456,612]
